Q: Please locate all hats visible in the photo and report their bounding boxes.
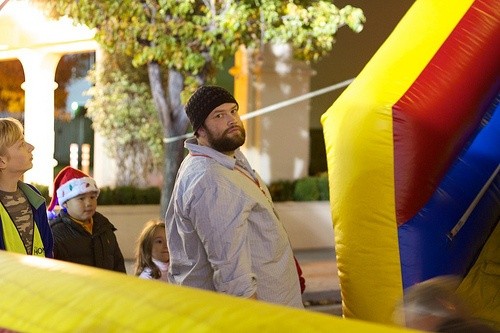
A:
[46,166,99,221]
[185,85,240,135]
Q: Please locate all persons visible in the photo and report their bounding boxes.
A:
[0,117,56,259]
[133,219,170,283]
[164,86,306,311]
[46,166,127,274]
